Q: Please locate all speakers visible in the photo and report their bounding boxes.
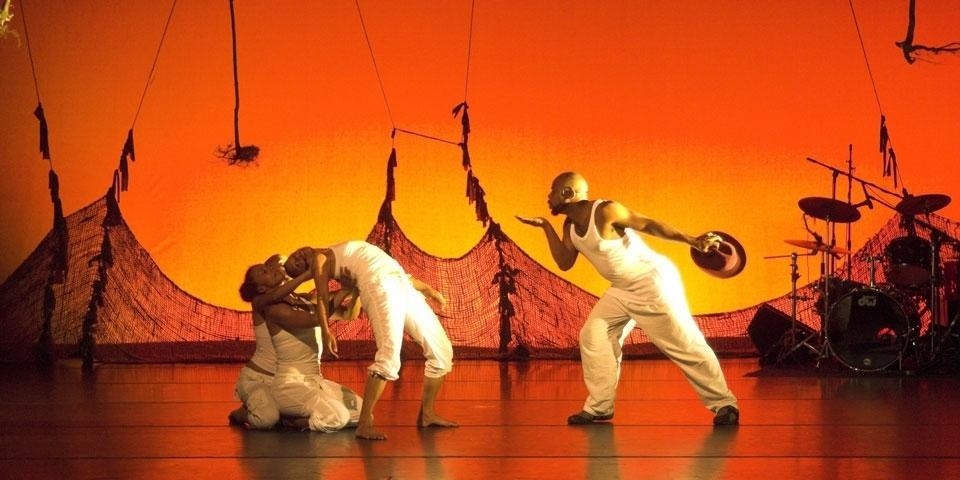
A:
[748,303,813,359]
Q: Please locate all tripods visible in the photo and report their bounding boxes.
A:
[788,250,833,370]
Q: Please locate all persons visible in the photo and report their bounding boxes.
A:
[228,250,352,432]
[514,170,741,427]
[283,238,460,442]
[238,261,372,434]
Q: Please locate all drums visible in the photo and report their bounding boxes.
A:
[885,236,941,291]
[825,285,922,372]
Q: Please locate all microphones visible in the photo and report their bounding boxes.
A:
[792,252,798,273]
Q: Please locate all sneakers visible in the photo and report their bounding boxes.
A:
[714,405,739,425]
[568,410,613,424]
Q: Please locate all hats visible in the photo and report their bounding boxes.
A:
[691,231,746,278]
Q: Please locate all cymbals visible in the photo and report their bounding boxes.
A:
[895,194,952,215]
[783,238,853,255]
[798,196,861,223]
